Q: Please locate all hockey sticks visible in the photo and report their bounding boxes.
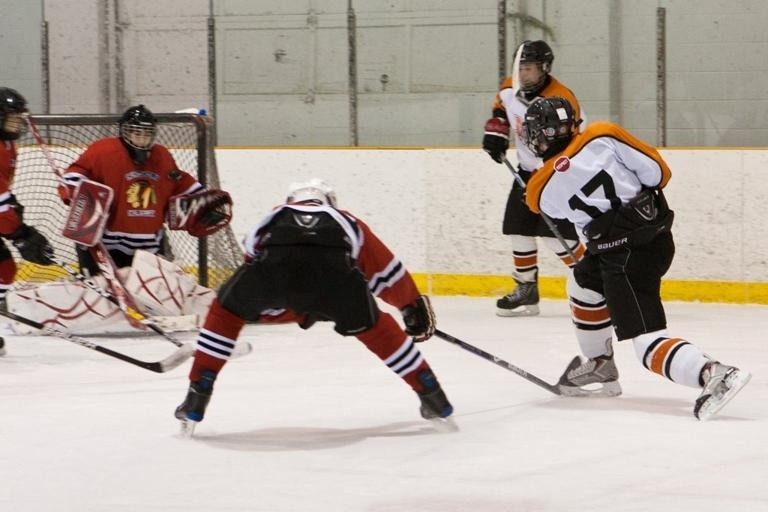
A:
[510,43,541,106]
[44,249,252,361]
[432,328,584,397]
[0,309,195,375]
[19,108,200,332]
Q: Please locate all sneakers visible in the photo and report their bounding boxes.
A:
[174,389,212,422]
[693,362,740,421]
[496,272,541,310]
[420,387,453,420]
[559,353,619,387]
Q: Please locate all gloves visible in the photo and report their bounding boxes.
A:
[479,133,509,165]
[15,227,56,266]
[402,296,437,344]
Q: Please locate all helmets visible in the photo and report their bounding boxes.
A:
[512,39,555,91]
[116,105,159,164]
[520,95,577,157]
[286,179,340,210]
[0,85,27,142]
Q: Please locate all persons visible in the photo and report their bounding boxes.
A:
[174,179,455,439]
[483,40,584,317]
[57,104,233,280]
[0,87,53,358]
[521,96,751,423]
